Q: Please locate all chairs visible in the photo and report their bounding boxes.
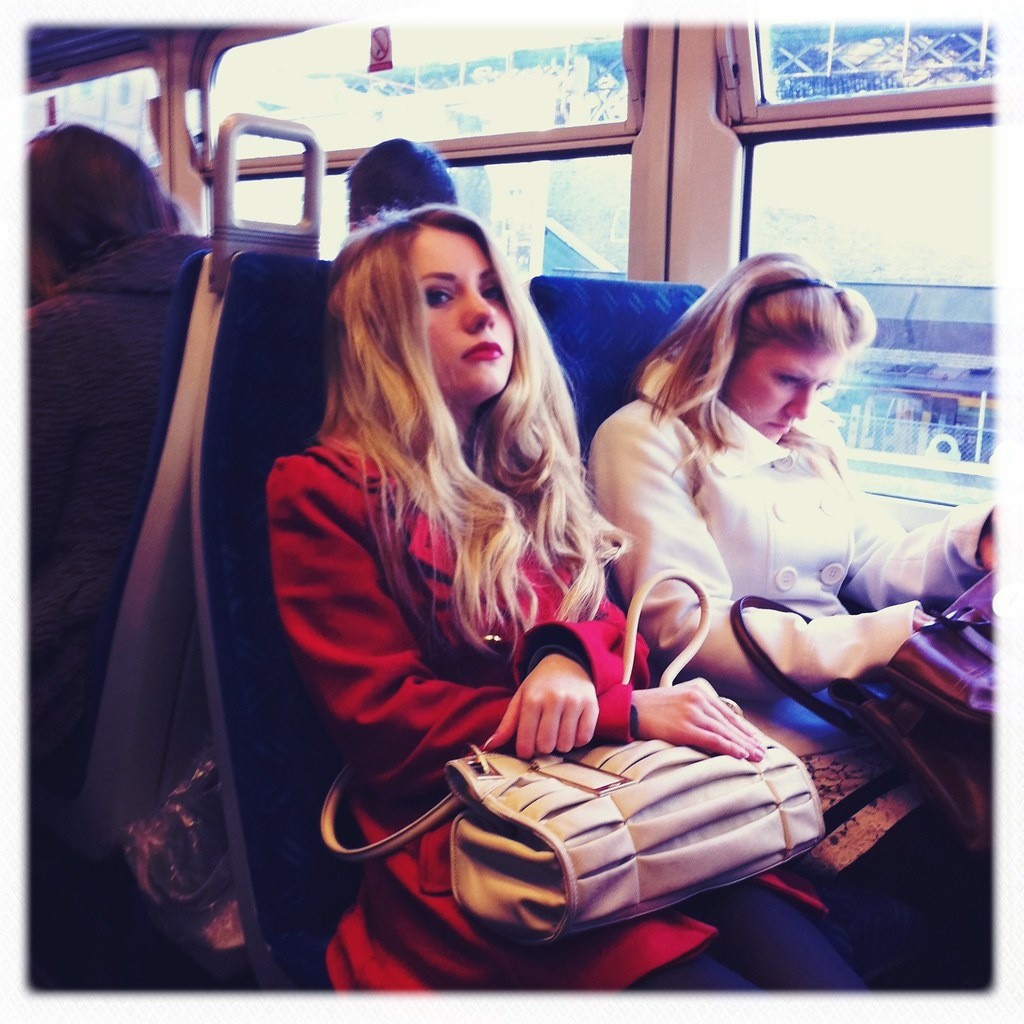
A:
[47,249,895,992]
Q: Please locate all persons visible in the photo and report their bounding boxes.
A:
[587,251,995,990]
[348,140,457,236]
[27,123,214,800]
[265,202,861,990]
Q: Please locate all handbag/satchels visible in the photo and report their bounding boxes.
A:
[730,569,992,857]
[319,570,827,944]
